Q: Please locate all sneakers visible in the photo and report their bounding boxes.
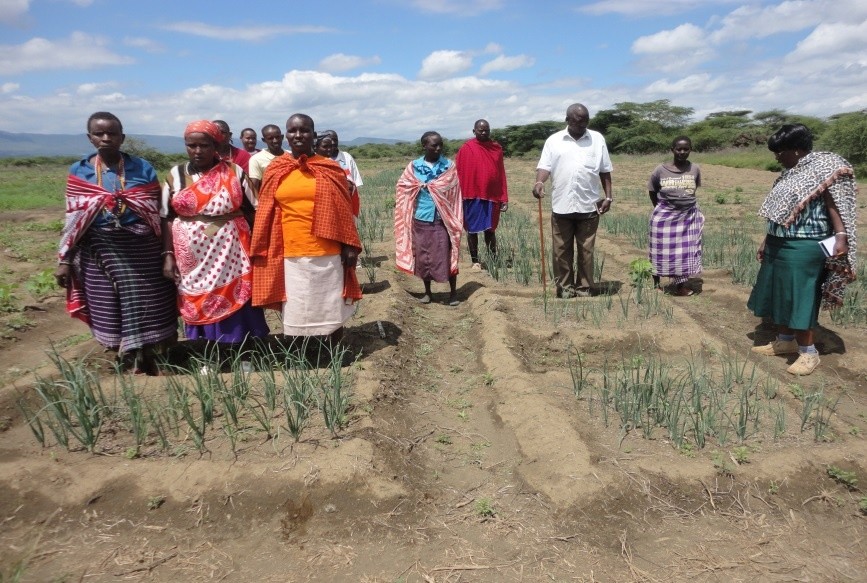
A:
[752,336,799,356]
[788,349,820,375]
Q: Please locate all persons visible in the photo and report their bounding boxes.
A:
[160,120,270,359]
[248,124,291,192]
[250,114,363,367]
[747,124,858,376]
[396,131,464,306]
[313,130,364,187]
[53,112,182,374]
[456,119,508,268]
[240,128,262,155]
[532,103,614,298]
[647,135,705,296]
[211,119,252,175]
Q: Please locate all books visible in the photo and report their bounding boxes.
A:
[818,236,837,257]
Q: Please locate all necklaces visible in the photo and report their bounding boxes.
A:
[95,154,125,224]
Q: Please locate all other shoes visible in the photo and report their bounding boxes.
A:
[239,360,255,373]
[157,362,166,376]
[472,263,481,273]
[126,363,142,374]
[200,363,217,375]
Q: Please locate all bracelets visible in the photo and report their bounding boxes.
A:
[161,251,173,255]
[536,182,542,184]
[59,262,70,265]
[836,231,847,235]
[606,198,613,202]
